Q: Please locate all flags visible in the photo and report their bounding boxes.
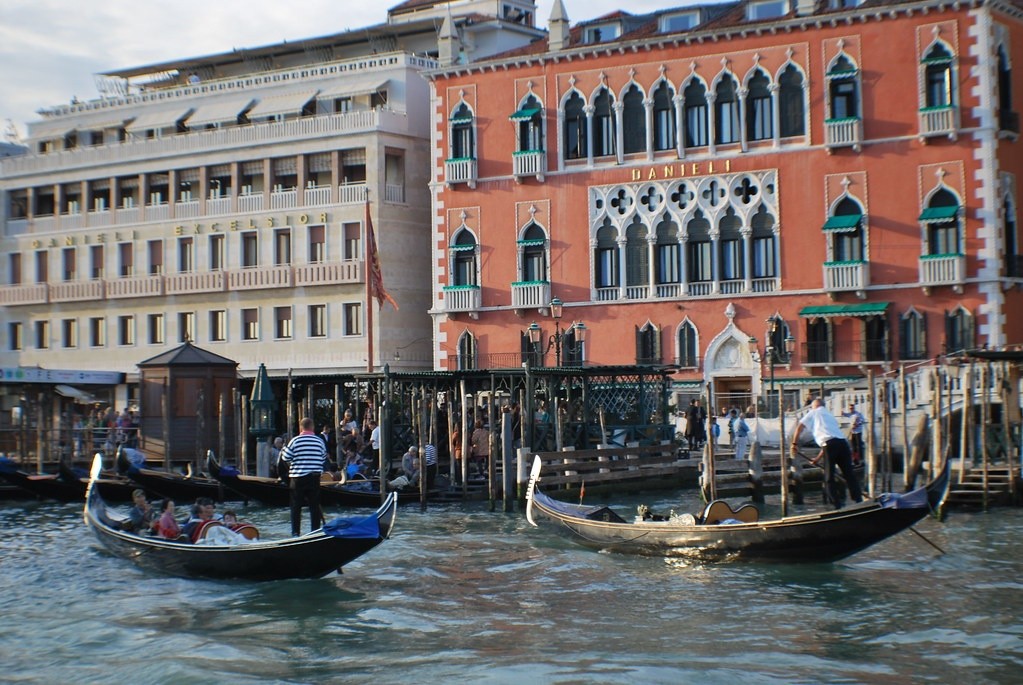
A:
[367,210,399,312]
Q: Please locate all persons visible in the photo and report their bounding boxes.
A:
[425,438,437,490]
[733,413,749,459]
[186,72,200,85]
[683,399,699,451]
[719,407,729,418]
[158,498,183,539]
[744,406,755,418]
[841,403,863,461]
[711,417,720,451]
[317,400,684,484]
[385,458,420,488]
[271,437,283,479]
[321,424,330,466]
[403,446,417,482]
[281,418,327,539]
[223,512,238,523]
[789,399,863,510]
[184,497,217,540]
[693,400,707,447]
[728,410,738,449]
[130,489,158,530]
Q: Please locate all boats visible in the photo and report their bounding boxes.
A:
[526,444,953,562]
[84,452,397,583]
[206,449,445,508]
[116,445,243,505]
[0,443,166,504]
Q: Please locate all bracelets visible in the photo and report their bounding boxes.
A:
[792,443,798,445]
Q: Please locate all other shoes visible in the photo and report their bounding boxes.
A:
[483,471,489,479]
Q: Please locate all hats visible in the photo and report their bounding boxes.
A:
[133,489,144,499]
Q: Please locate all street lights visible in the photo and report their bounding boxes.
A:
[748,313,796,418]
[528,296,586,406]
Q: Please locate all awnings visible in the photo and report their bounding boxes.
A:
[316,79,391,100]
[19,92,321,144]
[797,302,891,318]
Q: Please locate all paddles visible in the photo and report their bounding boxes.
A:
[139,468,217,481]
[796,451,946,554]
[318,504,343,574]
[26,475,57,480]
[237,474,388,483]
[80,477,134,483]
